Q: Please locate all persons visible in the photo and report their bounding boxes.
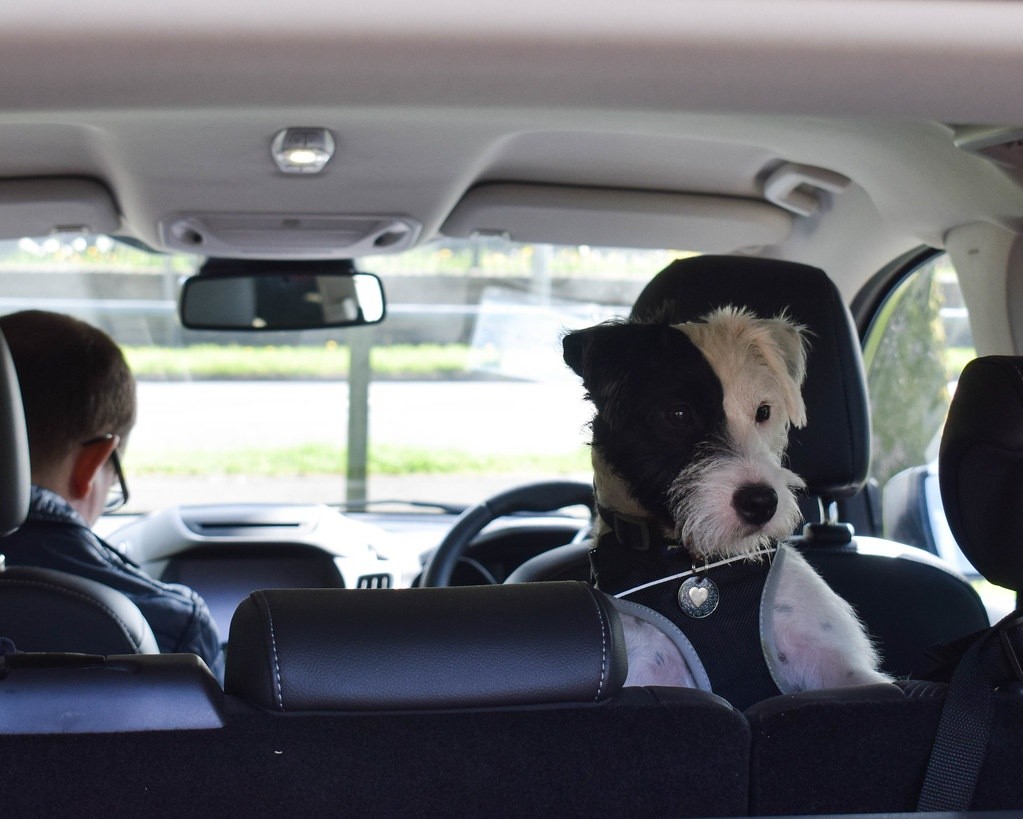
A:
[0,308,224,692]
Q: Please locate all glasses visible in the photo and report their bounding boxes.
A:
[81,434,129,513]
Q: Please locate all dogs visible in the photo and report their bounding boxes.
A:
[563,301,895,704]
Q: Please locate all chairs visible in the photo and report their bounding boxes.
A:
[0,256,1023,819]
